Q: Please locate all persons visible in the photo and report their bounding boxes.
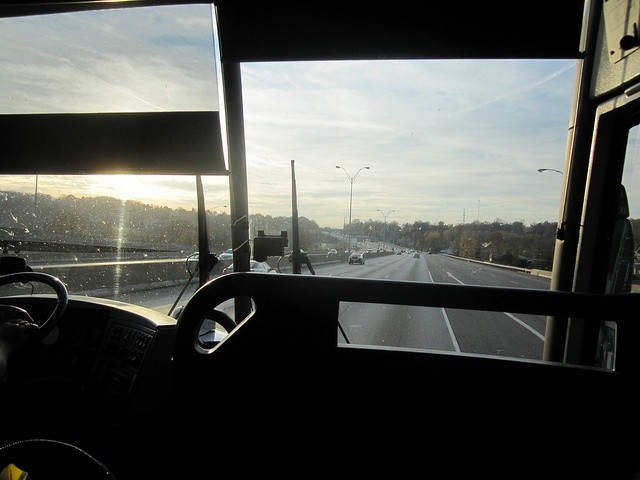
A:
[0,318,39,377]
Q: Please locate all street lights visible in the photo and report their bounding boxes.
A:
[336,165,370,253]
[537,167,564,175]
[207,205,228,249]
[377,209,396,250]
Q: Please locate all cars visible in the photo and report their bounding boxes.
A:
[327,248,373,255]
[396,250,420,259]
[348,253,365,265]
[222,259,277,274]
[219,249,234,260]
